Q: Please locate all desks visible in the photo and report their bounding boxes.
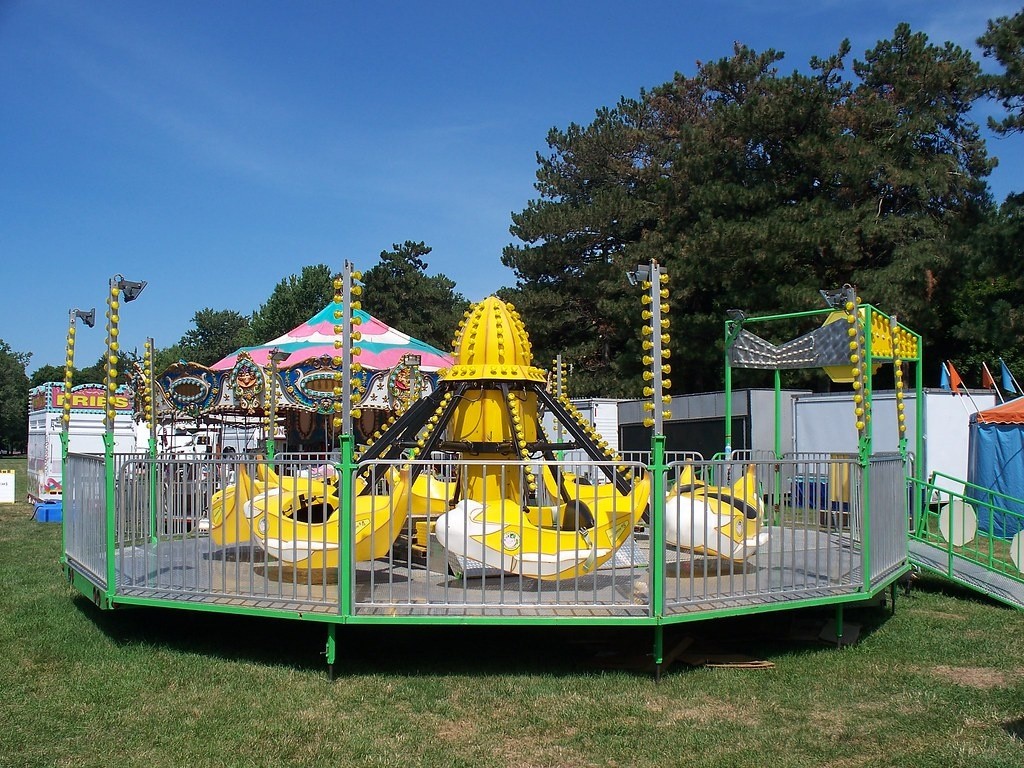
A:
[787,472,928,513]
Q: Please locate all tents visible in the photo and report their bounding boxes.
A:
[963,396,1024,538]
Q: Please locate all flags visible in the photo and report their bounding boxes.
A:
[982,367,993,392]
[940,365,950,392]
[948,362,961,396]
[1001,362,1016,393]
